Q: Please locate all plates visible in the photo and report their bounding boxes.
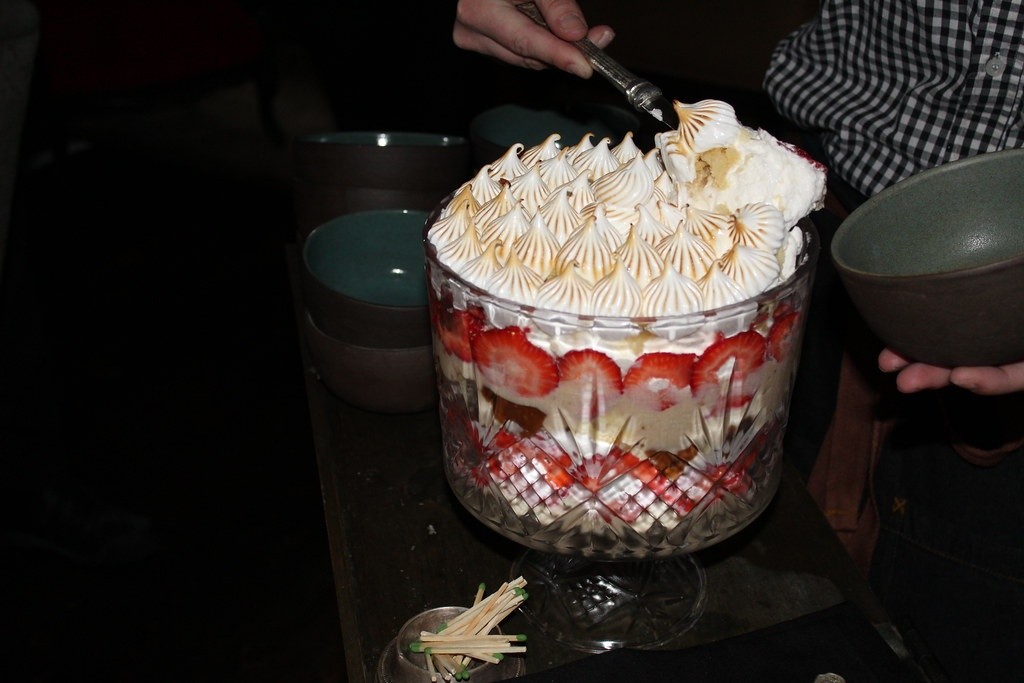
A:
[375,635,526,683]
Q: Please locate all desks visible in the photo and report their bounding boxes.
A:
[283,202,937,683]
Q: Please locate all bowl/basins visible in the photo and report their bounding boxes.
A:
[829,147,1024,369]
[297,128,468,417]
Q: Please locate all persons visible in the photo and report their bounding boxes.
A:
[452,0,1024,683]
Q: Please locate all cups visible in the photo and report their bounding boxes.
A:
[422,185,819,655]
[394,606,503,683]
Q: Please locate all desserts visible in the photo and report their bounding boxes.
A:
[426,98,826,538]
